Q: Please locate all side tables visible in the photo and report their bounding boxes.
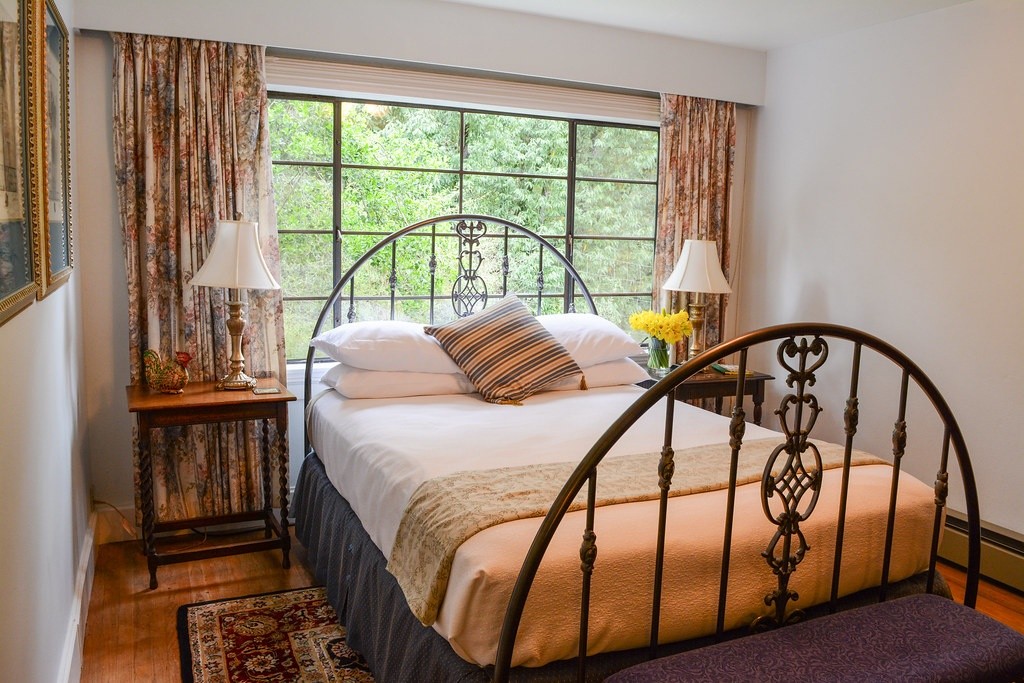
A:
[123,378,294,592]
[649,365,776,429]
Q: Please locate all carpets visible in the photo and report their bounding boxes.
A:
[176,582,383,682]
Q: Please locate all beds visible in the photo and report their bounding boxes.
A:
[293,383,950,681]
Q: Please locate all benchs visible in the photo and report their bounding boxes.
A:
[600,588,1024,683]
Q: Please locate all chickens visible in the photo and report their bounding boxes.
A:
[143,349,193,395]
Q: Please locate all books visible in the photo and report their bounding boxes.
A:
[713,362,754,376]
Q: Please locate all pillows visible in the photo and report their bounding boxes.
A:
[577,356,652,388]
[320,360,477,399]
[534,312,647,370]
[423,292,590,406]
[310,317,467,375]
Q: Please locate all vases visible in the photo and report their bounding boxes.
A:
[646,337,670,378]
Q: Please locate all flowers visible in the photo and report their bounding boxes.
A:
[629,308,694,345]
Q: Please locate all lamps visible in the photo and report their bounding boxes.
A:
[188,218,284,392]
[661,239,734,374]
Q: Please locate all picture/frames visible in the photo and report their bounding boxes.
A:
[25,0,74,301]
[0,1,37,328]
